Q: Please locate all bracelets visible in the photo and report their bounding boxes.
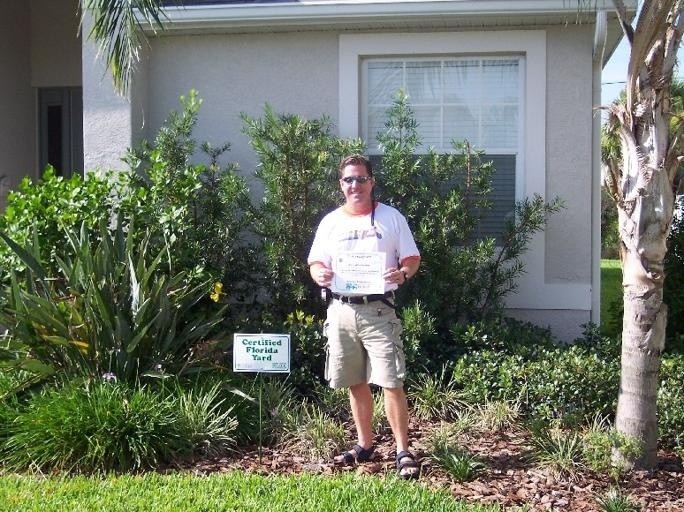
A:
[402,269,408,280]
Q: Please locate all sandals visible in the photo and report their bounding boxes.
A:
[330,444,377,465]
[394,449,421,479]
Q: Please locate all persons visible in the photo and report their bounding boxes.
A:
[307,153,424,479]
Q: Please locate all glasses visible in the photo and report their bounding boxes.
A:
[341,175,373,183]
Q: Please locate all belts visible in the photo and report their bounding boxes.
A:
[331,290,396,305]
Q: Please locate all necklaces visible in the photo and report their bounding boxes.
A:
[340,206,374,216]
